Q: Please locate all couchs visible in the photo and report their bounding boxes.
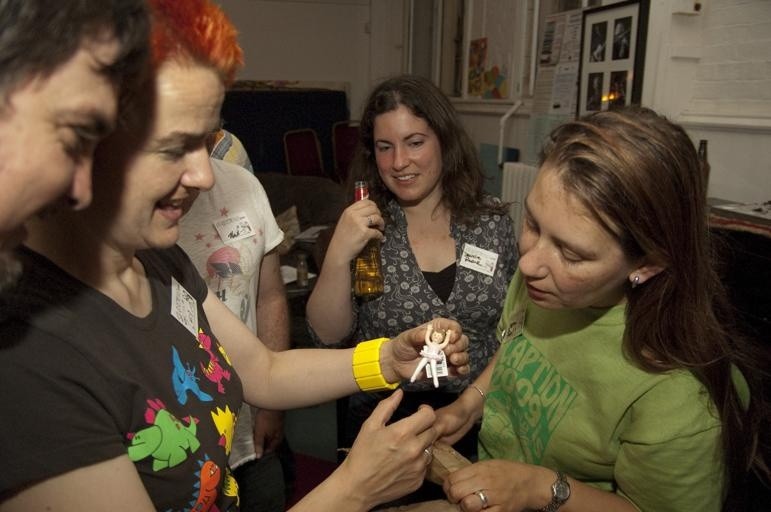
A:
[259,172,347,278]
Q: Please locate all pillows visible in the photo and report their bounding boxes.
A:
[274,205,302,257]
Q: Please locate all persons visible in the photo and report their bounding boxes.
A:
[173,154,290,512]
[0,0,472,511]
[431,105,771,512]
[295,73,520,502]
[0,1,150,287]
[209,126,255,174]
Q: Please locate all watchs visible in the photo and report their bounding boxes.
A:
[538,470,571,512]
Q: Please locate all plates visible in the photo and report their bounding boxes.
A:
[712,204,771,224]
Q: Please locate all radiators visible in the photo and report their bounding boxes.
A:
[499,162,537,244]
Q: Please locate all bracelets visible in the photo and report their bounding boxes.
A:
[352,337,402,393]
[468,384,484,396]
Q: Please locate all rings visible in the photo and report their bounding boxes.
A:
[474,490,488,508]
[425,449,432,466]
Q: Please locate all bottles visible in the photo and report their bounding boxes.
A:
[696,138,711,201]
[348,178,385,299]
[295,249,309,287]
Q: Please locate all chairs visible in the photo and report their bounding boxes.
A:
[331,118,364,184]
[282,125,327,179]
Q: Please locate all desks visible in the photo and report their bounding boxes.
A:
[281,264,318,302]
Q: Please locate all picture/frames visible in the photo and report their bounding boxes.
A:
[572,1,651,120]
